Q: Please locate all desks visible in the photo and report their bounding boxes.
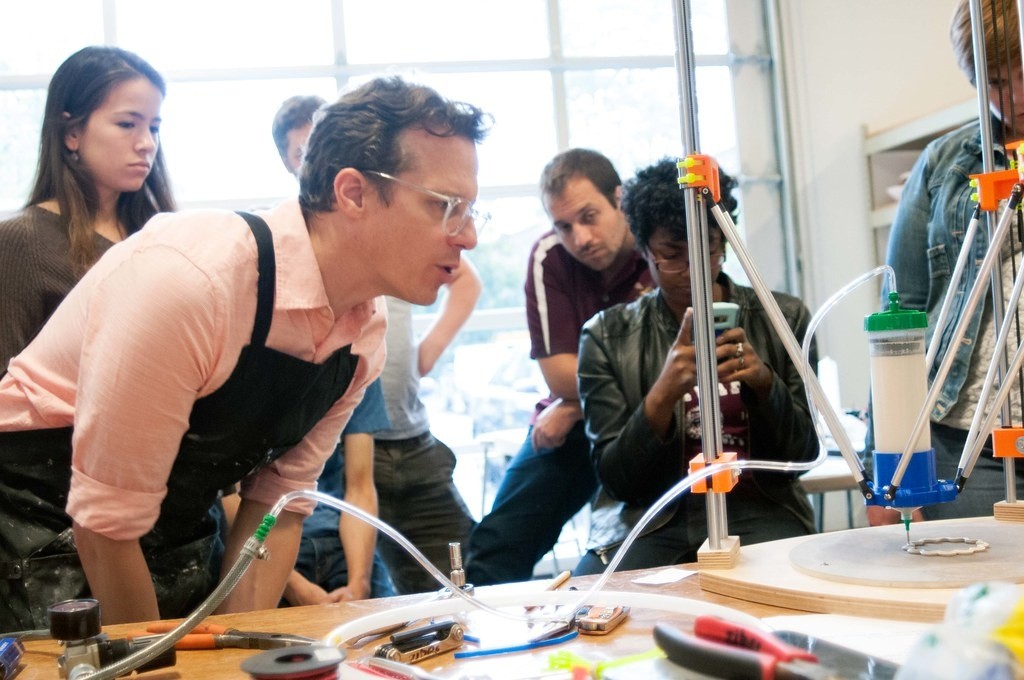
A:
[0,516,1024,680]
[798,455,855,534]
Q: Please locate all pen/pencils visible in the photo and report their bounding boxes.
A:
[545,569,573,590]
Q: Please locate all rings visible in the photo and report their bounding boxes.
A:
[736,343,743,357]
[741,358,744,369]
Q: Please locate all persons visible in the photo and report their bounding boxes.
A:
[456,147,657,587]
[0,77,485,633]
[860,0,1023,526]
[572,157,819,575]
[221,376,398,606]
[0,47,166,379]
[272,93,483,595]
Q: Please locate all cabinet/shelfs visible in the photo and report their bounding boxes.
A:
[863,93,981,302]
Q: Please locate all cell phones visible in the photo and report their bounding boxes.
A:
[712,302,741,365]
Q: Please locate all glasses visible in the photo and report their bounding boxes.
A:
[647,241,727,275]
[362,169,494,238]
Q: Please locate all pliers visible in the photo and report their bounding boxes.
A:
[125,621,319,650]
[651,615,836,680]
